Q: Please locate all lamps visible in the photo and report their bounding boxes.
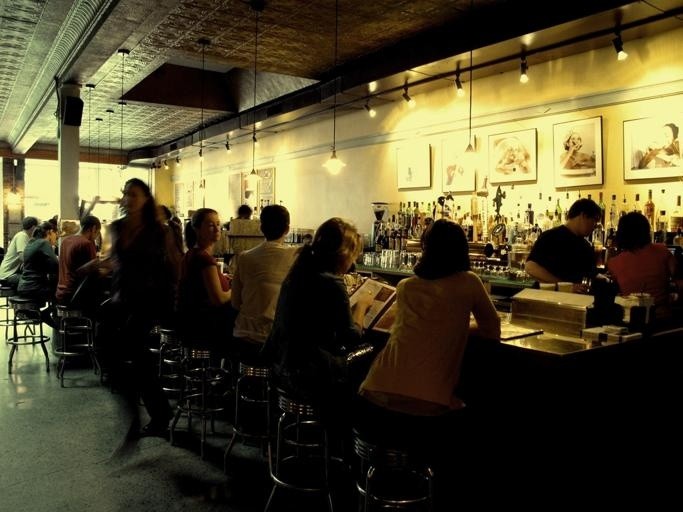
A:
[194,37,207,198]
[321,0,345,180]
[245,25,263,192]
[359,25,629,119]
[160,123,258,171]
[461,0,480,167]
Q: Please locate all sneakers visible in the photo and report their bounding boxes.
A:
[38,311,56,328]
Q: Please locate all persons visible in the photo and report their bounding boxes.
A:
[356,218,500,504]
[606,212,682,333]
[524,199,601,329]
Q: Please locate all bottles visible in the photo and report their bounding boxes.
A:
[364,183,683,283]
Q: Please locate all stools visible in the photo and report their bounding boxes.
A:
[157,329,433,512]
[0,286,103,389]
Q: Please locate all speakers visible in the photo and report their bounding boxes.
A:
[15,159,25,180]
[63,96,84,126]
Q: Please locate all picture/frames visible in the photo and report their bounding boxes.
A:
[438,136,476,194]
[486,128,538,184]
[622,115,682,184]
[551,115,603,190]
[394,142,431,190]
